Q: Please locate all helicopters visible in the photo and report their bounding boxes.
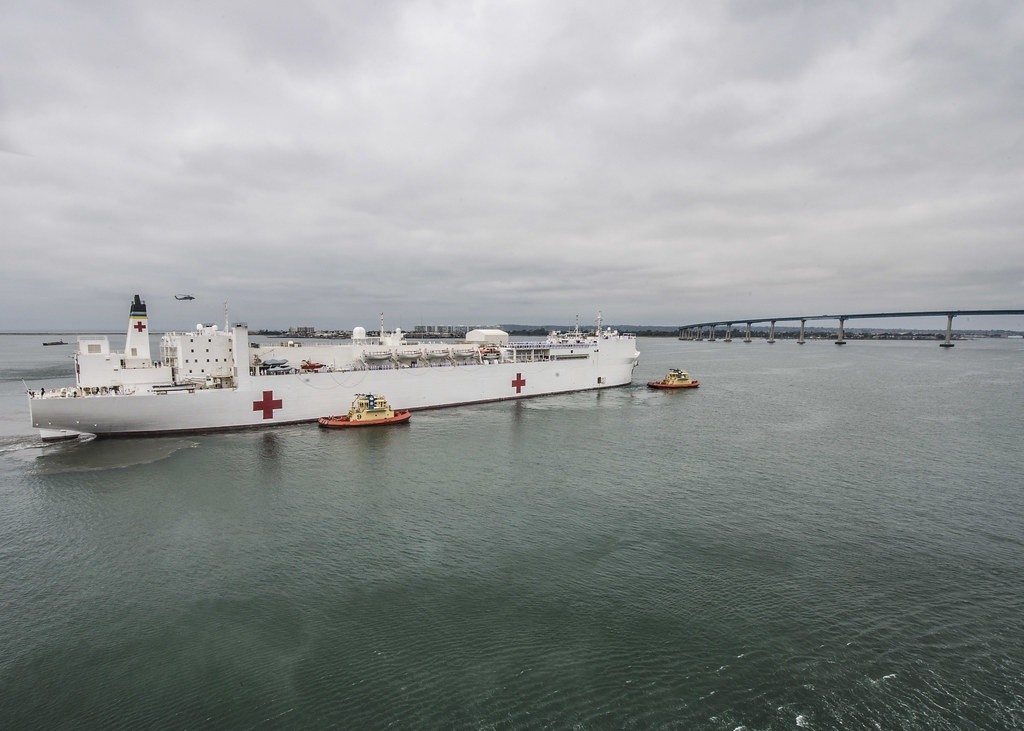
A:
[174,295,195,301]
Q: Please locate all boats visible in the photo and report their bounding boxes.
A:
[42,339,68,346]
[318,392,412,428]
[647,367,700,388]
[21,294,640,436]
